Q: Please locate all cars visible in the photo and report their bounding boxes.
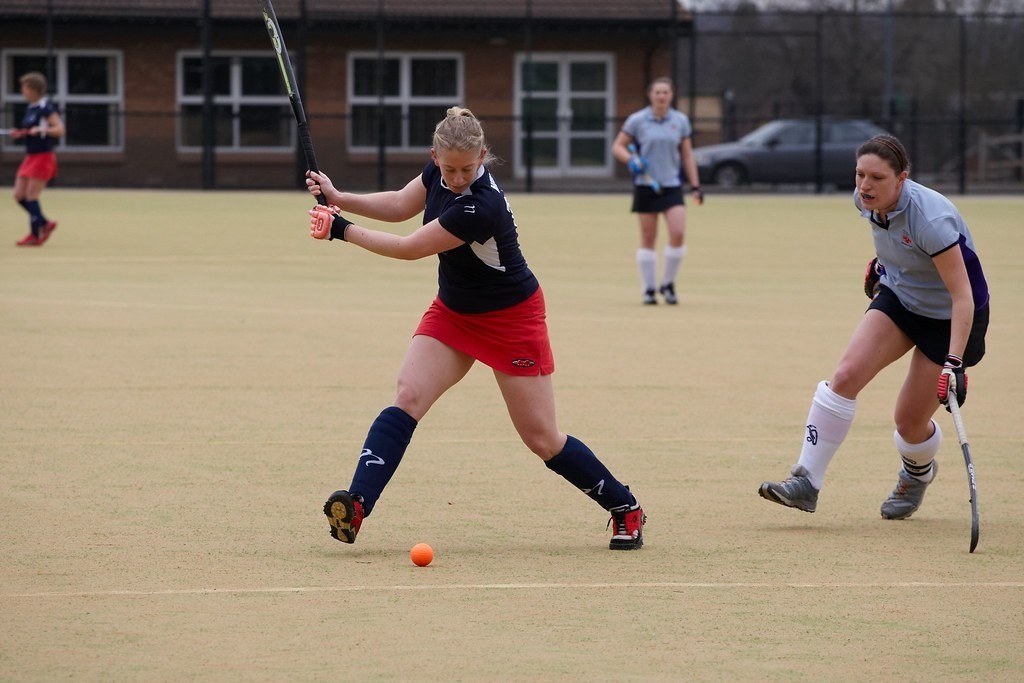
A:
[692,119,890,185]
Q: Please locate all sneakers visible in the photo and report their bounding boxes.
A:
[323,490,363,543]
[758,465,819,513]
[606,485,647,549]
[880,459,937,519]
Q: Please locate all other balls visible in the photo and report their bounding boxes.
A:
[409,542,434,567]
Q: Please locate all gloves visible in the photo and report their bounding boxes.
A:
[309,204,353,242]
[626,157,646,176]
[690,186,704,205]
[864,256,885,299]
[937,353,969,411]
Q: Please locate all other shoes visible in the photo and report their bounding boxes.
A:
[16,233,40,245]
[660,282,677,303]
[35,221,57,244]
[642,288,656,304]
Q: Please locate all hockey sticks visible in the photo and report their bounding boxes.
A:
[260,0,337,229]
[949,391,982,554]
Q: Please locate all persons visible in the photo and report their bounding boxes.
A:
[12,73,65,247]
[612,77,704,306]
[757,133,989,522]
[305,107,647,553]
